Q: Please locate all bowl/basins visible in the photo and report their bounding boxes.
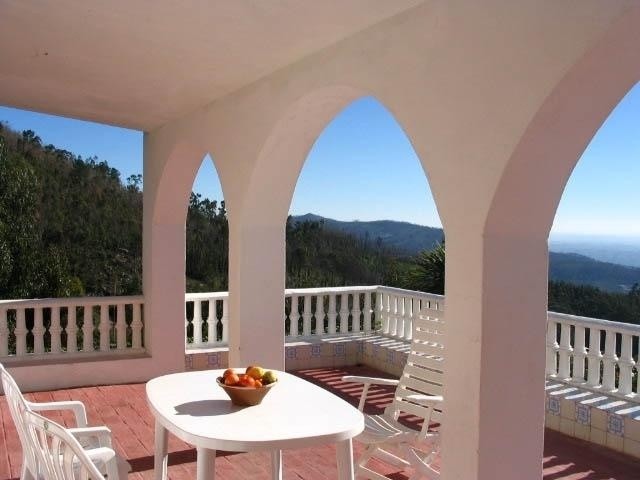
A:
[216,373,279,407]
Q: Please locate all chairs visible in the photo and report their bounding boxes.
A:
[0,362,132,480]
[336,307,443,480]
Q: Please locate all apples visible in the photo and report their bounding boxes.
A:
[224,366,277,387]
